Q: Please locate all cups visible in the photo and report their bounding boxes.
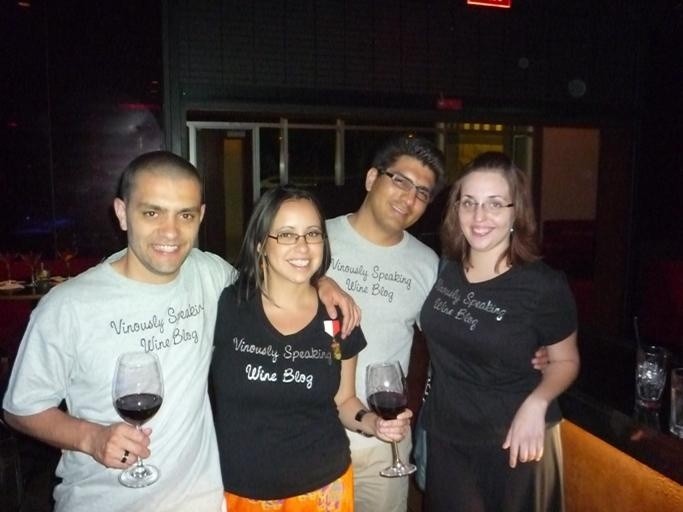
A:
[453,200,516,213]
[267,228,329,246]
[381,169,437,206]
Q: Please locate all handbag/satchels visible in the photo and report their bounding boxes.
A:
[355,409,376,438]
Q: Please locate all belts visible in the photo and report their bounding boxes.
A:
[112,353,164,488]
[366,362,418,480]
[1,230,77,289]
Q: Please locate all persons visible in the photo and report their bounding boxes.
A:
[0,150,363,512]
[410,149,582,511]
[207,181,413,512]
[321,138,549,511]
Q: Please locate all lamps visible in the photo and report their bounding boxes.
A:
[637,253,683,356]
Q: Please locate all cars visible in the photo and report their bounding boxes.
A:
[635,345,683,439]
